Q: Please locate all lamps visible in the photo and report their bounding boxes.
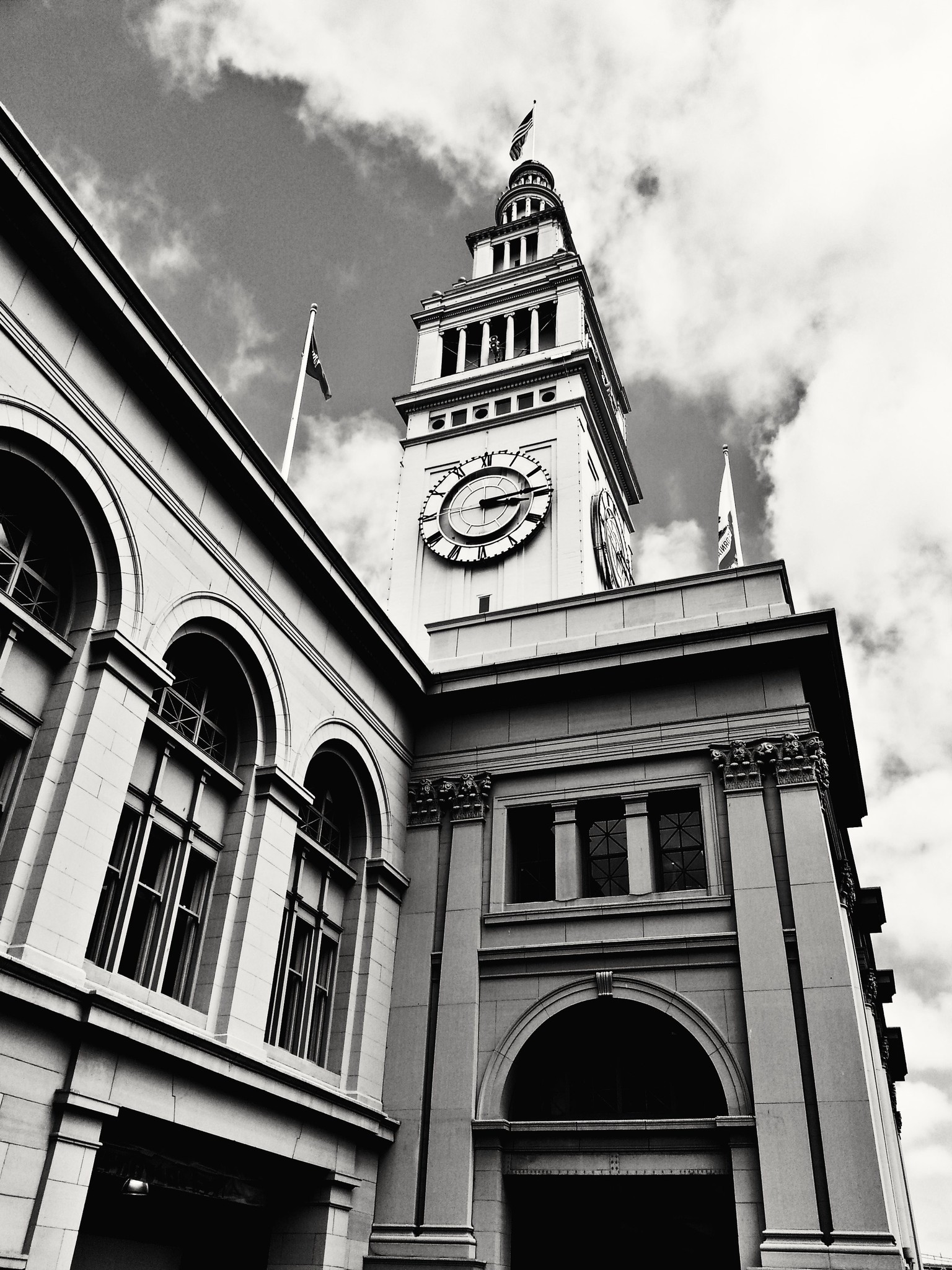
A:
[122,1178,149,1196]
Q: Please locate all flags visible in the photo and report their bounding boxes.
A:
[717,459,740,572]
[510,107,533,162]
[302,321,335,401]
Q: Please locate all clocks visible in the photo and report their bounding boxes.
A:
[592,489,635,589]
[418,450,554,562]
